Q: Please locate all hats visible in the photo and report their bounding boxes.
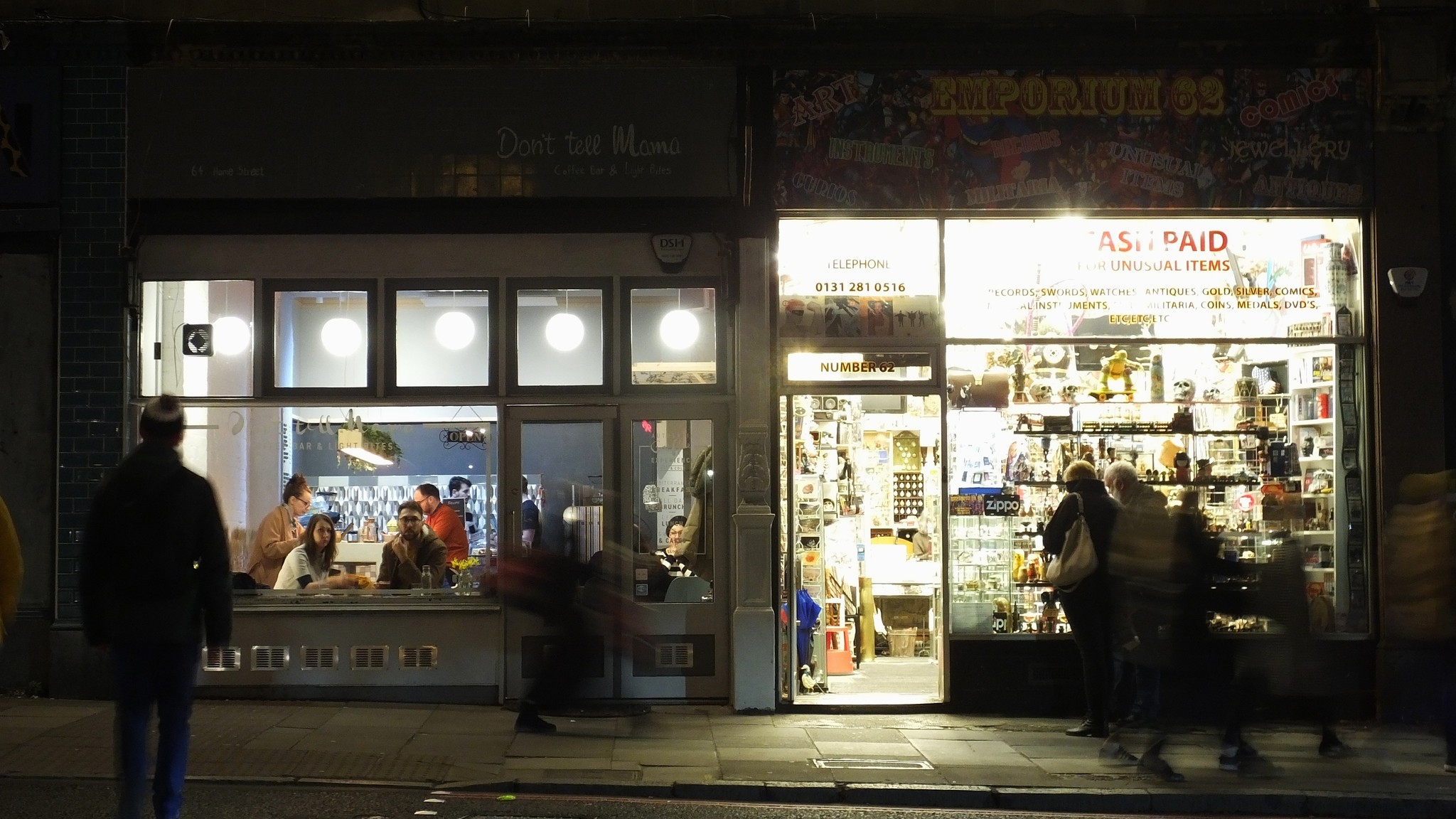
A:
[140,394,184,445]
[666,516,687,537]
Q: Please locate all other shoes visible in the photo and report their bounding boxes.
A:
[1220,748,1269,769]
[1065,717,1109,738]
[1099,746,1140,765]
[1444,752,1456,771]
[1318,736,1345,756]
[1136,755,1184,784]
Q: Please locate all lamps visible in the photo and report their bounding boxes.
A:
[660,289,700,351]
[321,292,363,357]
[436,293,474,352]
[544,290,585,353]
[211,280,250,355]
[642,421,663,513]
[337,406,396,466]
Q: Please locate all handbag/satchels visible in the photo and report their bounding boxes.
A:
[1045,493,1097,585]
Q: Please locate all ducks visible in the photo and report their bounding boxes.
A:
[801,665,820,693]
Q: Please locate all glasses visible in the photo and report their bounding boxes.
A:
[417,496,432,506]
[294,496,310,509]
[398,515,422,525]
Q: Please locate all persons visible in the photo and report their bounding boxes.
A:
[246,474,312,589]
[377,501,448,589]
[1042,460,1174,737]
[1133,492,1353,783]
[0,496,25,639]
[414,484,469,585]
[77,394,233,819]
[449,476,476,534]
[1389,473,1456,772]
[521,477,539,549]
[657,515,696,582]
[273,514,370,589]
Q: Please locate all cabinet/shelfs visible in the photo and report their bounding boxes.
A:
[1013,399,1261,587]
[794,418,865,694]
[949,514,1012,604]
[1286,342,1336,608]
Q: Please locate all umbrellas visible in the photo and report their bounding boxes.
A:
[782,566,821,691]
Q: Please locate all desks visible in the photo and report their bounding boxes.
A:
[334,542,388,575]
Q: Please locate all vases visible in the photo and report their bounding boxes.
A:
[457,568,474,596]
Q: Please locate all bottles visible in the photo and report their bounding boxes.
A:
[421,565,432,596]
[362,518,376,543]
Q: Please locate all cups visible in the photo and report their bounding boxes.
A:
[321,584,329,595]
[410,583,421,596]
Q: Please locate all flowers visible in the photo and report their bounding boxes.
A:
[450,555,479,594]
